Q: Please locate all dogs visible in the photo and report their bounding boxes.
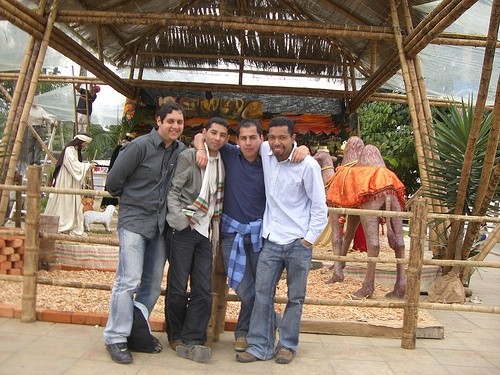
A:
[83,206,118,232]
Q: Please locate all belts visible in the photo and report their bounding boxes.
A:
[32,125,43,127]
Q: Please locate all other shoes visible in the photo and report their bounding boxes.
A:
[176,344,212,363]
[235,337,248,352]
[170,338,187,352]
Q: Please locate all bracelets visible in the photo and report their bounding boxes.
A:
[195,148,206,151]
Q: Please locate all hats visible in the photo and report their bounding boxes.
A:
[93,86,100,92]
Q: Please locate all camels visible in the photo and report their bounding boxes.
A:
[313,136,407,299]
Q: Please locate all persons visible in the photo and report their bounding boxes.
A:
[165,117,327,364]
[44,133,97,236]
[75,83,100,134]
[104,100,185,363]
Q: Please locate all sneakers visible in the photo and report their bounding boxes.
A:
[274,347,293,363]
[236,352,257,362]
[106,342,132,363]
[127,339,162,353]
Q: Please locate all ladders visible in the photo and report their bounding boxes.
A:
[70,64,95,202]
[4,118,66,224]
